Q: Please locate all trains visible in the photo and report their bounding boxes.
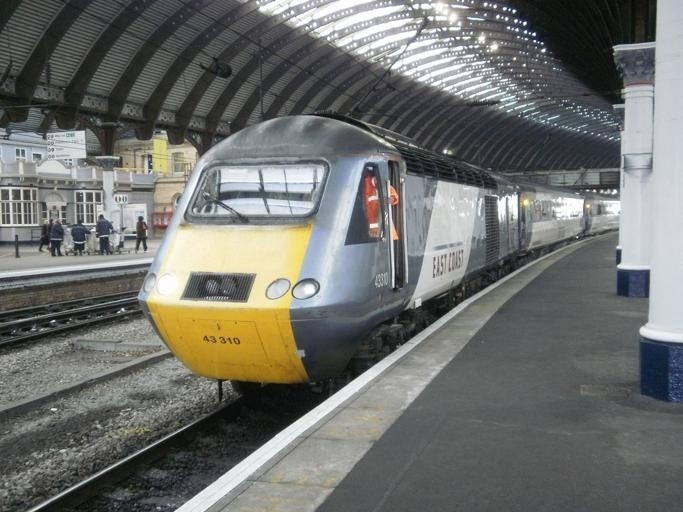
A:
[136,109,619,405]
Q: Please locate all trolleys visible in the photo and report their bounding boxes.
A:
[86,232,102,255]
[109,231,131,254]
[64,234,85,255]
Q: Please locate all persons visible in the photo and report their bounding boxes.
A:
[70,219,91,256]
[96,214,113,255]
[362,168,401,292]
[135,215,148,254]
[37,219,64,257]
